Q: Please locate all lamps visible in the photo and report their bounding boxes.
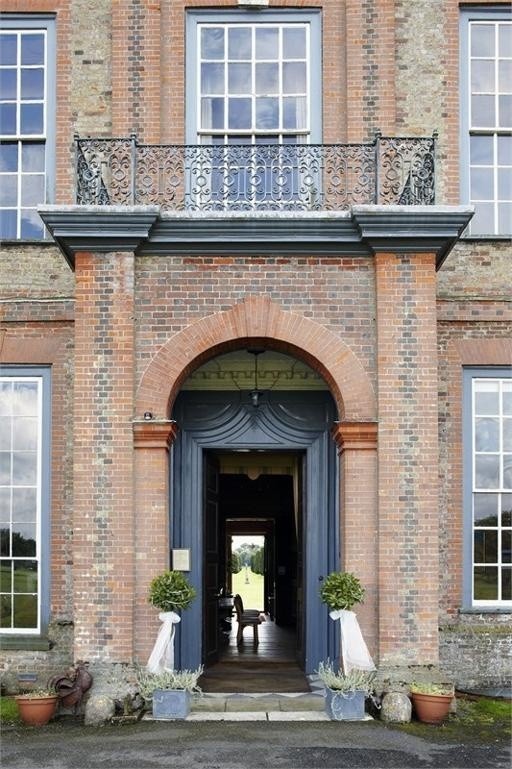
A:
[246,346,266,407]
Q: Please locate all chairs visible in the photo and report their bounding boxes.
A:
[233,593,261,648]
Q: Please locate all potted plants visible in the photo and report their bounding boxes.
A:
[135,663,206,719]
[314,658,377,721]
[145,570,197,672]
[411,680,456,725]
[319,570,378,674]
[14,687,59,727]
